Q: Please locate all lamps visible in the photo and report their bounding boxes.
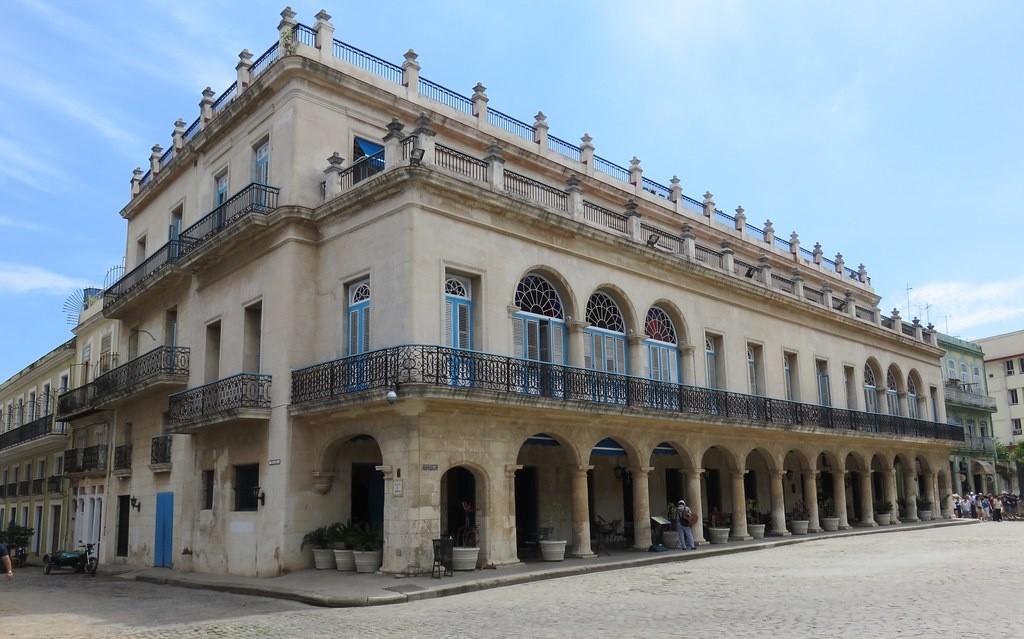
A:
[745,267,757,279]
[130,494,140,512]
[251,480,265,505]
[786,468,794,481]
[614,465,624,482]
[409,148,426,167]
[646,234,660,248]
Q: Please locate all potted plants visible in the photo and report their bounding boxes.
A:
[746,497,766,539]
[447,500,481,569]
[790,499,814,535]
[181,547,193,572]
[331,519,360,570]
[707,507,730,544]
[941,494,953,519]
[300,522,341,569]
[347,520,386,573]
[660,502,683,549]
[820,498,840,531]
[875,498,896,526]
[538,498,568,561]
[916,493,932,521]
[0,524,36,574]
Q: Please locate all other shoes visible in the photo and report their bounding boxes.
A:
[683,548,686,550]
[691,548,696,549]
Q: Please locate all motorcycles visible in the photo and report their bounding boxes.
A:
[41,540,98,575]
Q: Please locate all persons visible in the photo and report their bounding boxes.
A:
[673,500,697,550]
[952,491,1024,523]
[0,543,13,581]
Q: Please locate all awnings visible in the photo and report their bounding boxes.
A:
[355,136,384,168]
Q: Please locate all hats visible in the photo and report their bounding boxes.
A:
[678,500,685,505]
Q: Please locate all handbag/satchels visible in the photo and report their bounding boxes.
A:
[683,510,698,525]
[680,517,692,528]
